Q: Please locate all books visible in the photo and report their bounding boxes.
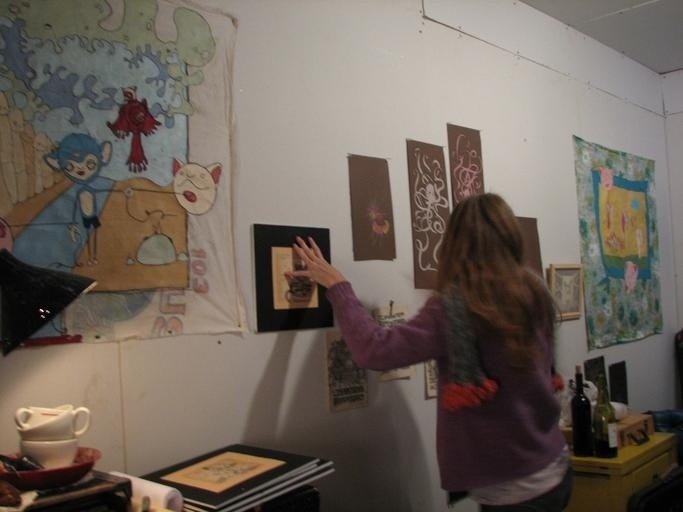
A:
[139,444,336,512]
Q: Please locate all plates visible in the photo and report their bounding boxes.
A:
[1,446,101,491]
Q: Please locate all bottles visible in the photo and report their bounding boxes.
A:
[571,372,618,458]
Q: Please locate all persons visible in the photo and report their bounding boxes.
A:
[285,194,574,512]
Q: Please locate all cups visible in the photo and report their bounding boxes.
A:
[14,404,91,468]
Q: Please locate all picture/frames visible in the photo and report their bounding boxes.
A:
[254,223,334,333]
[139,443,335,512]
[550,263,583,322]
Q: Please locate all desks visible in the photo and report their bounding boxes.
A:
[27,470,132,512]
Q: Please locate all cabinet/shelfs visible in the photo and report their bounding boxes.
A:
[565,432,678,512]
[244,484,322,512]
